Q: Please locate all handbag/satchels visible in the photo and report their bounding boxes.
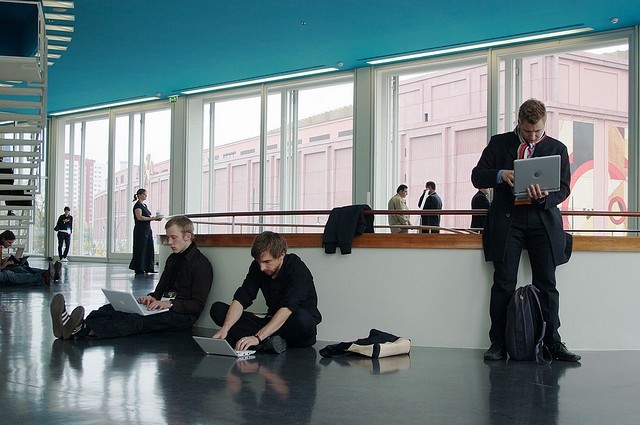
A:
[319,329,411,359]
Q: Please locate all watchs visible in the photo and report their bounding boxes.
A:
[170,300,174,309]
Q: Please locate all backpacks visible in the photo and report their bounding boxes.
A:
[504,284,553,366]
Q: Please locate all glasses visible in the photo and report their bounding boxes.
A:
[6,240,13,244]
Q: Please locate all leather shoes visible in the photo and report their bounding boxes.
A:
[262,335,287,354]
[145,270,158,273]
[135,270,144,274]
[543,342,581,362]
[483,341,507,360]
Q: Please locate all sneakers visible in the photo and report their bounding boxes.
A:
[55,261,61,281]
[65,257,68,262]
[60,257,66,262]
[45,265,55,285]
[62,306,87,340]
[50,294,71,338]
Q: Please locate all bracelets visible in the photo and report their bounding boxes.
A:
[254,333,261,344]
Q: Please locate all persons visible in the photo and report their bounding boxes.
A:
[0,155,16,215]
[209,231,322,355]
[388,184,412,234]
[54,206,73,262]
[49,215,214,341]
[0,263,56,286]
[0,230,62,281]
[128,188,163,274]
[470,99,582,362]
[417,181,441,233]
[470,187,489,228]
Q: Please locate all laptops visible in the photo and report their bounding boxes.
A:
[101,288,169,316]
[14,247,25,259]
[192,336,256,357]
[512,155,561,196]
